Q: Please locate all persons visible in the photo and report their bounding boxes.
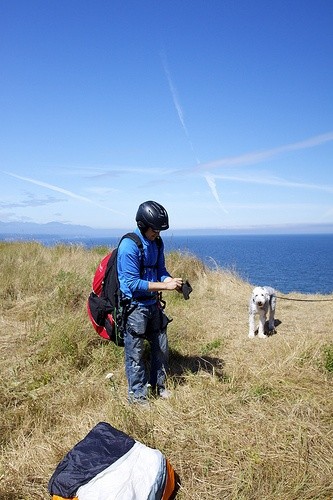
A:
[115,200,183,403]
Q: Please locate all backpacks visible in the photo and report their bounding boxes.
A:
[87,232,163,347]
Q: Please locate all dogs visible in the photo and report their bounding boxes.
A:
[248,286,277,339]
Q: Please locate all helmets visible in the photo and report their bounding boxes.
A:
[135,201,170,230]
